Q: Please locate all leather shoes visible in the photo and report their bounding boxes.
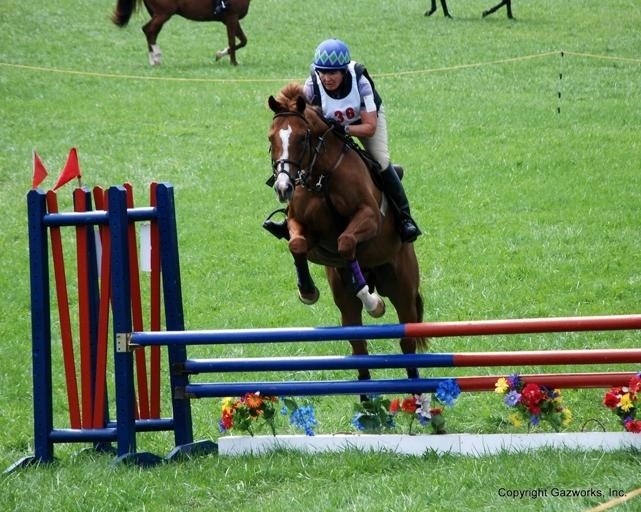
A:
[262,217,291,242]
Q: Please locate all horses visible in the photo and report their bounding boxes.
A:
[424,0,513,21]
[265,82,434,409]
[109,0,252,66]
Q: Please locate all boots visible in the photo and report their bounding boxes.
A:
[376,162,422,240]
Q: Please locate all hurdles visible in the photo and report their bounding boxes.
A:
[2,177,641,470]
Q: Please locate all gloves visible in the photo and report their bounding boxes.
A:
[326,117,347,134]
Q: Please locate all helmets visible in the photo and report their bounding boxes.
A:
[311,38,353,71]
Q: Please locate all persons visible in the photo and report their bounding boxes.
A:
[261,37,419,244]
